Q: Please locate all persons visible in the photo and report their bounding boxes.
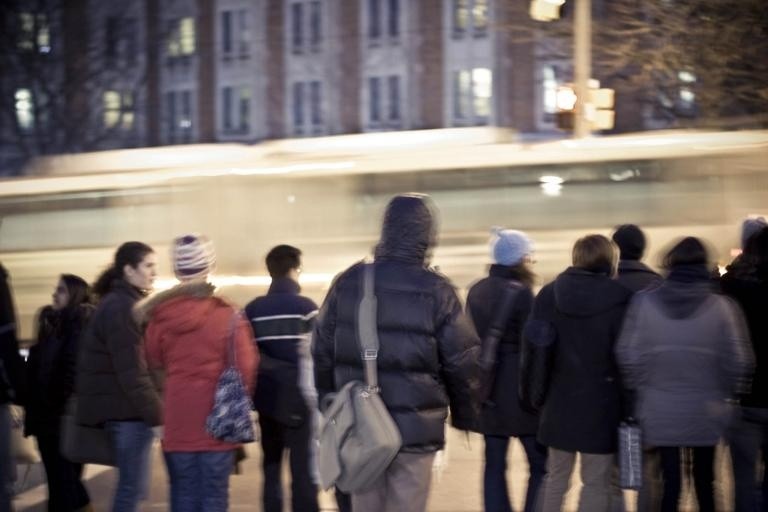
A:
[613,233,756,511]
[241,243,325,511]
[711,212,768,512]
[308,191,482,512]
[18,272,97,511]
[516,232,636,511]
[606,220,661,293]
[463,226,551,512]
[77,240,165,511]
[133,232,261,511]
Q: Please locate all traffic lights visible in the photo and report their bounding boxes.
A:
[554,87,578,131]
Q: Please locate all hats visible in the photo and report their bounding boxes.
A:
[492,228,535,266]
[173,234,215,282]
[741,217,767,244]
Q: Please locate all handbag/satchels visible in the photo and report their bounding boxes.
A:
[61,399,120,470]
[618,423,644,490]
[205,370,254,445]
[316,380,403,494]
[8,403,42,466]
